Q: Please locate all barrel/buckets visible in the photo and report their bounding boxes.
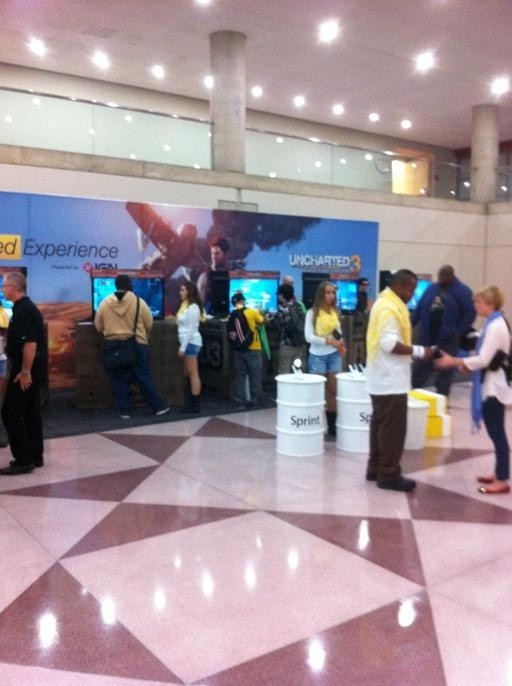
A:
[404,397,430,451]
[334,373,373,454]
[274,372,327,456]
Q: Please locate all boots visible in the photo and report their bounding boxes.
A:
[179,396,200,413]
[326,411,336,435]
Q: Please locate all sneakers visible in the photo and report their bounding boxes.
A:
[477,477,509,493]
[119,412,130,419]
[366,472,415,491]
[155,406,170,416]
[0,461,43,475]
[232,397,258,405]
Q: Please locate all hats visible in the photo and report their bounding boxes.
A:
[232,293,246,305]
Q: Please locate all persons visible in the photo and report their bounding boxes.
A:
[0,305,10,447]
[365,268,436,492]
[196,236,244,314]
[231,292,265,409]
[410,265,477,396]
[357,278,369,312]
[283,275,294,286]
[303,280,347,436]
[264,283,309,403]
[0,271,50,475]
[94,274,170,420]
[431,283,511,494]
[176,282,204,414]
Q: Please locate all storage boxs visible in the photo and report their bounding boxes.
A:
[428,416,454,438]
[407,387,449,414]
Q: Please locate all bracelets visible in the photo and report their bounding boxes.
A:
[411,345,426,360]
[21,369,32,374]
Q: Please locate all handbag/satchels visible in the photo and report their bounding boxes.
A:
[103,336,139,372]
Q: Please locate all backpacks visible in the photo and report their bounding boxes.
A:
[284,302,306,347]
[227,307,253,351]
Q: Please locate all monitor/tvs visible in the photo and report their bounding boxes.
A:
[0,274,14,320]
[406,278,432,312]
[305,278,359,314]
[93,277,166,320]
[223,277,281,319]
